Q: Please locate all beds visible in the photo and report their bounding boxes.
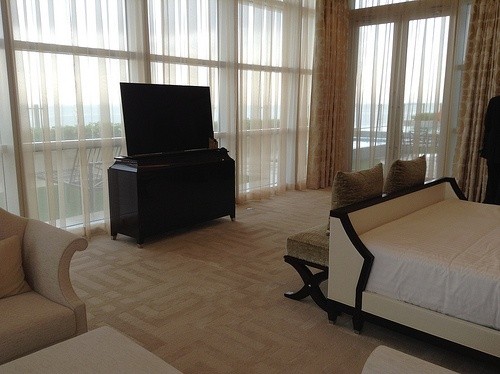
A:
[327,176,500,360]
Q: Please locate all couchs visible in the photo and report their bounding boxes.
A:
[0,208,88,365]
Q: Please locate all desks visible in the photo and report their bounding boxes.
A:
[0,325,184,374]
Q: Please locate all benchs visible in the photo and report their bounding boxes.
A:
[283,226,329,314]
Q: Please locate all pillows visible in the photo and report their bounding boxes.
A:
[0,235,32,299]
[386,155,426,195]
[326,163,383,235]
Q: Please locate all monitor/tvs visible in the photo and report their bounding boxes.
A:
[120,82,215,158]
